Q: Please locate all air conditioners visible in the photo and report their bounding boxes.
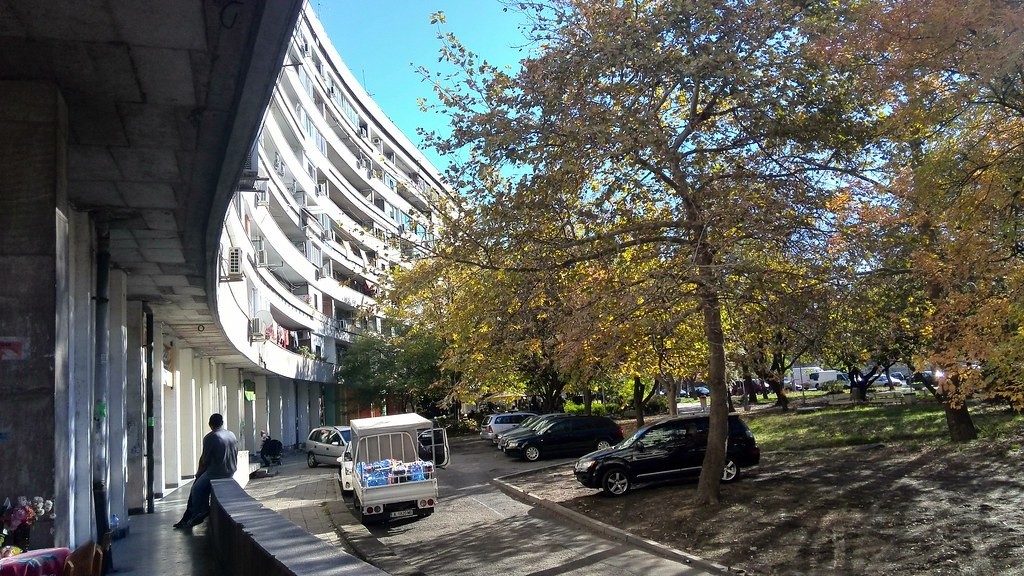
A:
[256,42,405,341]
[252,316,267,340]
[228,248,245,280]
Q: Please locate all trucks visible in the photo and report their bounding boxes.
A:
[352,428,451,524]
[793,367,851,389]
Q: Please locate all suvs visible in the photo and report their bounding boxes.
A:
[574,412,761,498]
[493,412,625,462]
[304,425,351,469]
[479,411,538,440]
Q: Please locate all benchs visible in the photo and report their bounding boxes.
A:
[828,393,858,408]
[866,391,904,407]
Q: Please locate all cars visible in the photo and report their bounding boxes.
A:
[726,377,803,395]
[660,386,710,397]
[336,440,353,493]
[858,370,943,387]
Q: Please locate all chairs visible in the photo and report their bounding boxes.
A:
[62,539,104,576]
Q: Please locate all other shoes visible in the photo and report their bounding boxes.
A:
[173,520,187,528]
[187,510,210,527]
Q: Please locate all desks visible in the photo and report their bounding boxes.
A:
[0,546,71,576]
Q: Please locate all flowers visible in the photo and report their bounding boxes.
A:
[0,493,56,535]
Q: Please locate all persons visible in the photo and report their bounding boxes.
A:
[260,430,271,466]
[174,413,238,528]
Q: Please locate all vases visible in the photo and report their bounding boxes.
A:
[4,521,30,553]
